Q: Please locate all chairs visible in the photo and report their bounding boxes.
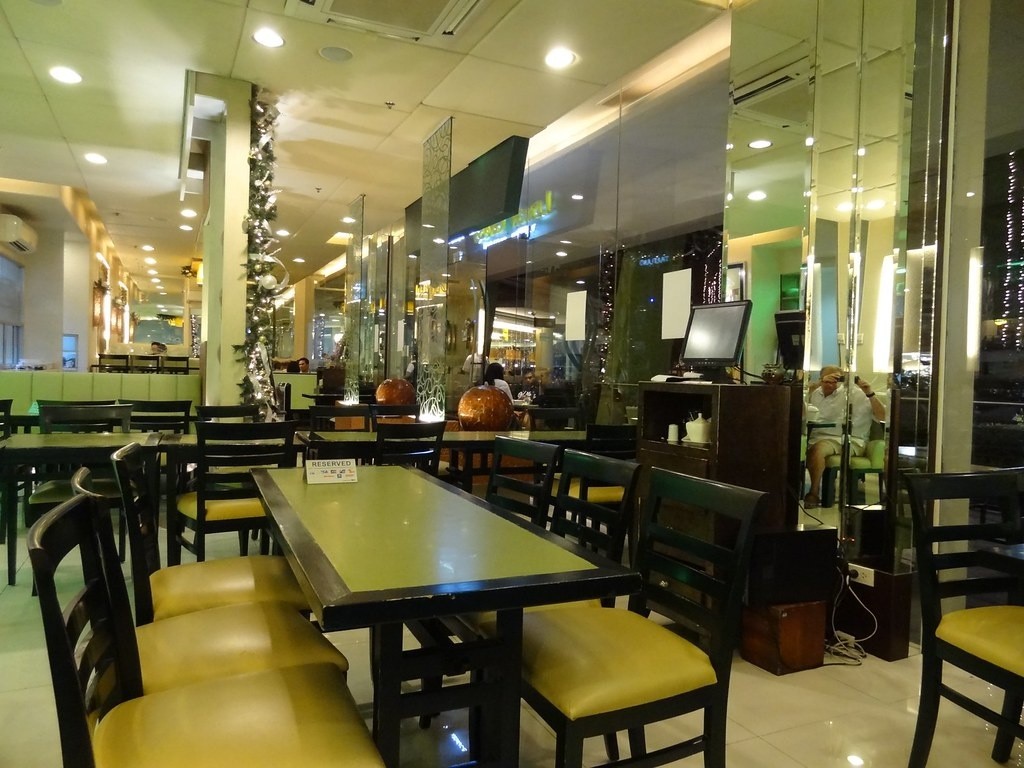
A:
[531,409,635,553]
[904,467,1023,768]
[484,466,766,768]
[27,493,387,767]
[822,421,887,509]
[111,440,315,628]
[0,396,445,586]
[419,437,640,759]
[71,467,349,707]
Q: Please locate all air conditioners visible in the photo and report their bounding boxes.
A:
[0,211,40,257]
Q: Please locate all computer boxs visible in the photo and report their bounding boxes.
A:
[746,525,838,605]
[844,505,886,559]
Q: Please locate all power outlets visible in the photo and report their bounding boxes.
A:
[847,563,875,589]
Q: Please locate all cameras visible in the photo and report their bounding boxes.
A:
[836,376,859,384]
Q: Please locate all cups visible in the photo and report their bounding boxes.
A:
[686,423,711,442]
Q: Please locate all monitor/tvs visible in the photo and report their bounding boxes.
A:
[679,299,753,380]
[775,310,807,385]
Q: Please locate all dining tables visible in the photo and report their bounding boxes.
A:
[250,464,647,768]
[4,429,305,586]
[294,430,630,497]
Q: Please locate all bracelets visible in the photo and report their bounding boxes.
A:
[865,392,875,398]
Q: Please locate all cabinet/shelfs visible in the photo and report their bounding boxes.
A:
[636,381,794,653]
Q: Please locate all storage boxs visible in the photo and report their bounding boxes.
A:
[741,600,827,677]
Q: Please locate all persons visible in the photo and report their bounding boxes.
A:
[482,363,514,404]
[286,360,300,374]
[149,342,168,355]
[516,367,540,402]
[298,357,311,374]
[404,352,417,388]
[461,341,491,386]
[804,363,887,509]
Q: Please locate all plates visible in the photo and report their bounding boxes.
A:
[682,439,711,446]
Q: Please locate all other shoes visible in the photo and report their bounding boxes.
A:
[803,493,819,509]
